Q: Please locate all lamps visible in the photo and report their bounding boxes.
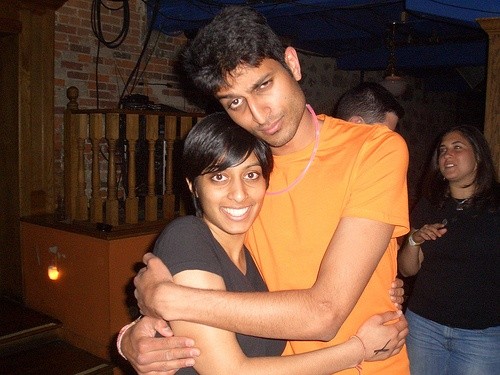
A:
[376,21,410,98]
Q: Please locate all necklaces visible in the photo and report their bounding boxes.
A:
[256,100,320,195]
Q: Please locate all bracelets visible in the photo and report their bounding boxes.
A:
[115,313,142,361]
[349,334,366,373]
[406,229,425,247]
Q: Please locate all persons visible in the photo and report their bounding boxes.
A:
[109,8,414,374]
[153,112,409,375]
[336,82,405,133]
[397,125,500,375]
